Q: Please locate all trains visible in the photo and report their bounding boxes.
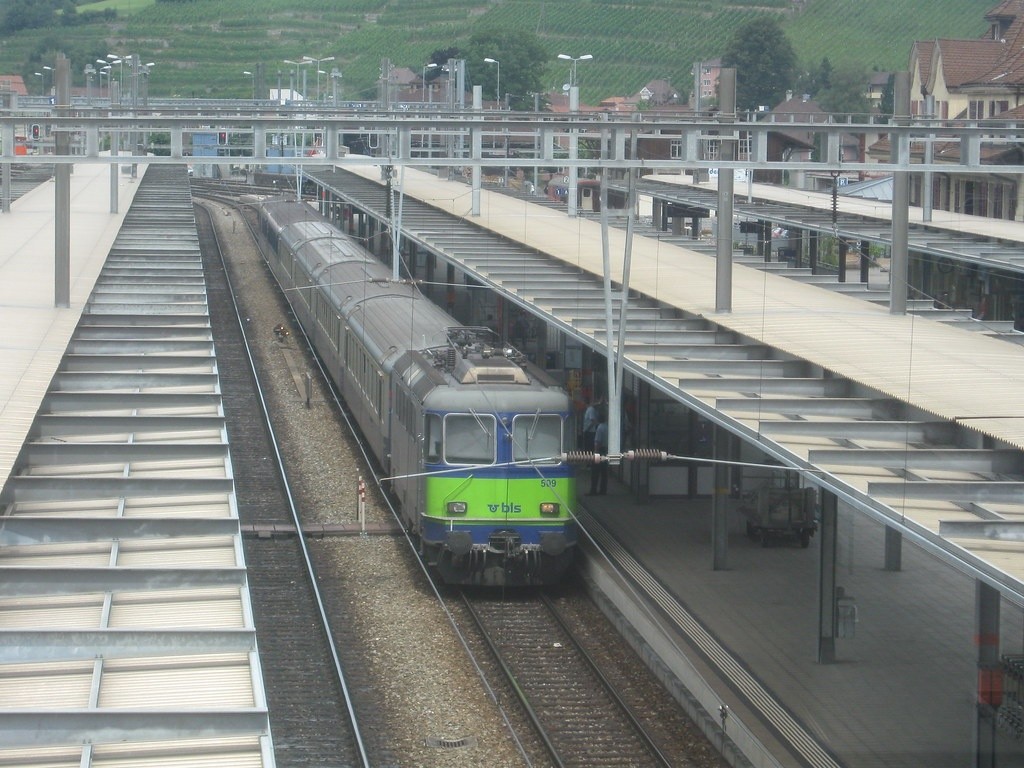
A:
[255,191,582,589]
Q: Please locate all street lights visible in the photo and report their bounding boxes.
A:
[483,57,500,110]
[302,56,335,100]
[283,60,313,118]
[34,72,44,102]
[557,53,593,215]
[96,53,155,108]
[43,66,56,104]
[318,70,329,101]
[244,71,255,100]
[422,63,437,102]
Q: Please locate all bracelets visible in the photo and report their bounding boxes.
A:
[581,431,583,433]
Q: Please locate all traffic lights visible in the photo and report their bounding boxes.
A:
[31,124,40,140]
[217,131,227,146]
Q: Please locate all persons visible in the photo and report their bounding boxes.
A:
[585,415,609,494]
[578,398,597,465]
[482,314,496,331]
